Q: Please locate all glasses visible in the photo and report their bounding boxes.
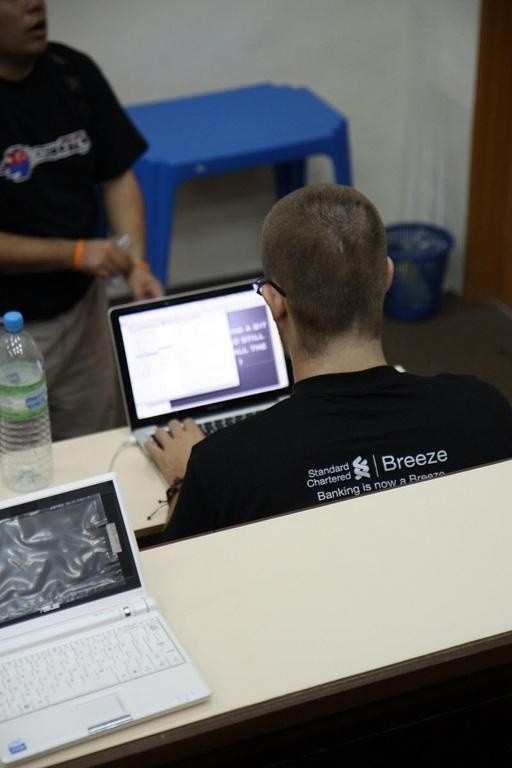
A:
[253,275,290,299]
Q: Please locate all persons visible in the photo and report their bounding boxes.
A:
[144,182,512,545]
[2,0,168,448]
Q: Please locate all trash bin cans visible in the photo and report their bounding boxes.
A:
[384,224,452,321]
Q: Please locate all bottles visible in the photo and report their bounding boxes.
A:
[0,310,55,495]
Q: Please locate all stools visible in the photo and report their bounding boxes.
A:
[122,81,354,291]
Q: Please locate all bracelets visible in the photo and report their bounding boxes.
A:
[147,473,186,524]
[122,252,148,263]
[73,234,88,275]
[126,262,152,282]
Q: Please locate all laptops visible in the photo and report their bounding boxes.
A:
[0,473,211,767]
[107,277,294,459]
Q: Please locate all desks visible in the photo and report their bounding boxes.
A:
[1,365,407,539]
[1,460,512,768]
